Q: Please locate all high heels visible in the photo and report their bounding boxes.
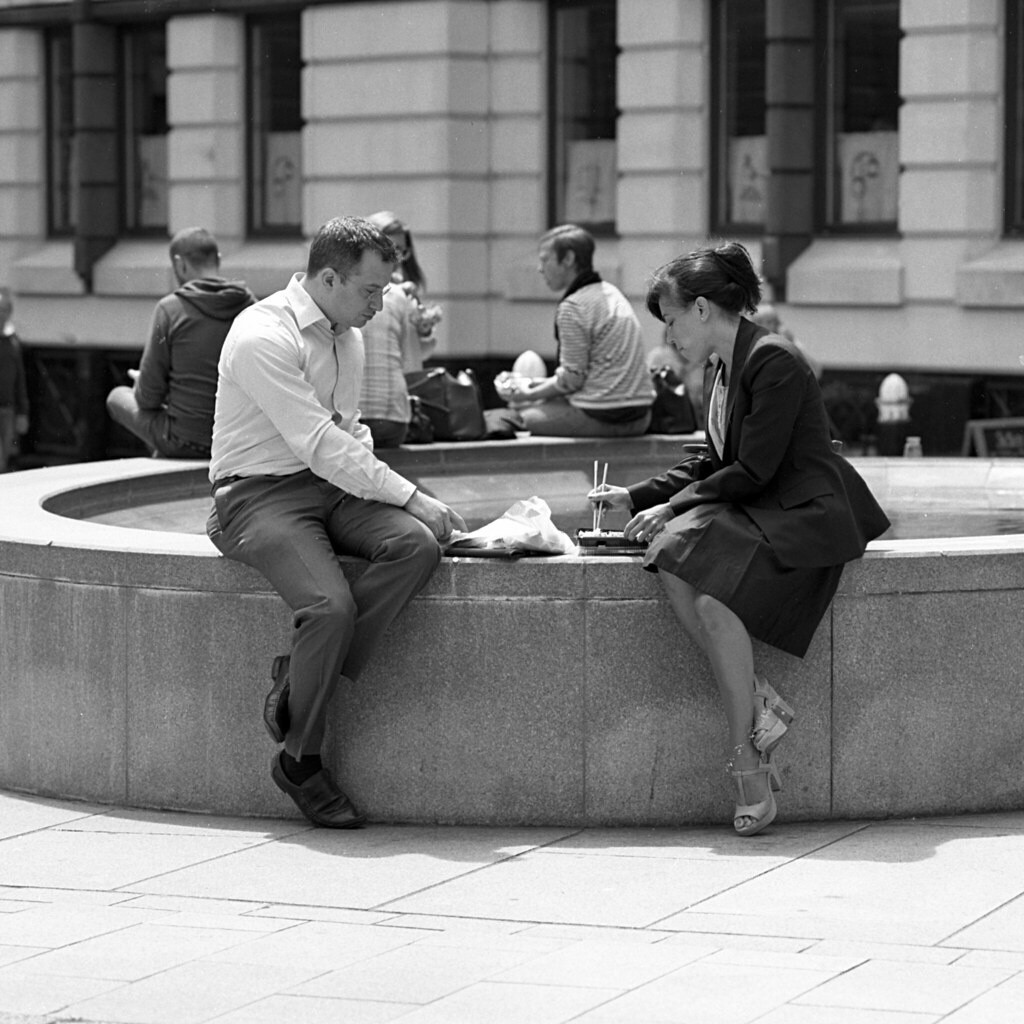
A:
[752,671,796,755]
[731,753,783,835]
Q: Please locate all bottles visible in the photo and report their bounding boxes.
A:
[904,437,923,457]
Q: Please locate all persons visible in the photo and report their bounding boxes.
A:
[107,228,260,460]
[0,286,28,473]
[647,304,821,434]
[498,225,658,438]
[350,211,444,449]
[205,215,469,828]
[589,242,891,839]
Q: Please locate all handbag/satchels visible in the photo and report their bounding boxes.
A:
[403,368,487,444]
[650,365,696,434]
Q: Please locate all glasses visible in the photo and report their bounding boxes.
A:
[343,273,392,300]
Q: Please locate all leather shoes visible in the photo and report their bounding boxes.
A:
[264,656,291,744]
[270,750,359,827]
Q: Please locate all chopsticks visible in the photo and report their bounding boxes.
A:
[593,460,608,528]
